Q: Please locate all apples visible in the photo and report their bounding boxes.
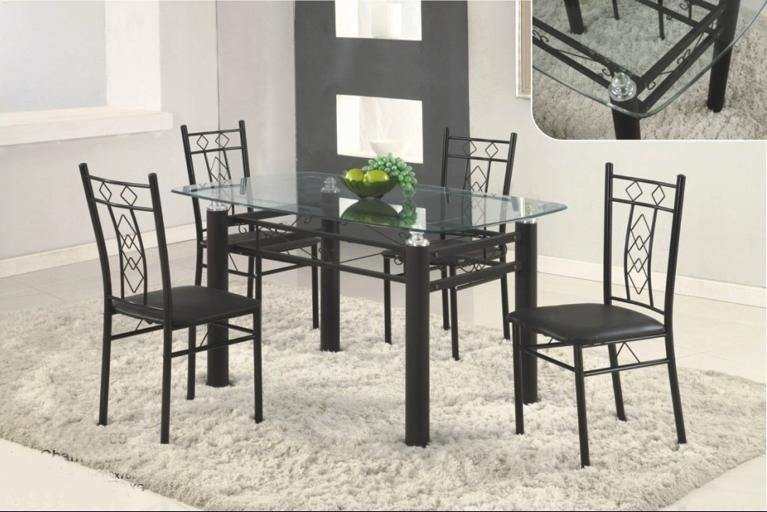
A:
[345,168,390,182]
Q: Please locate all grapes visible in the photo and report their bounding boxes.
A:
[398,199,417,225]
[361,154,418,198]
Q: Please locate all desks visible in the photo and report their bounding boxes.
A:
[527,0,767,142]
[169,169,573,452]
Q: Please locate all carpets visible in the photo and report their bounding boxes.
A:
[1,282,766,512]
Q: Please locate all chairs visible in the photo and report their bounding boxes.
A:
[76,158,265,446]
[177,120,323,331]
[378,125,519,362]
[506,160,691,471]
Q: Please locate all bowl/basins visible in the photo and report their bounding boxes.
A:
[335,174,396,200]
[339,199,400,223]
